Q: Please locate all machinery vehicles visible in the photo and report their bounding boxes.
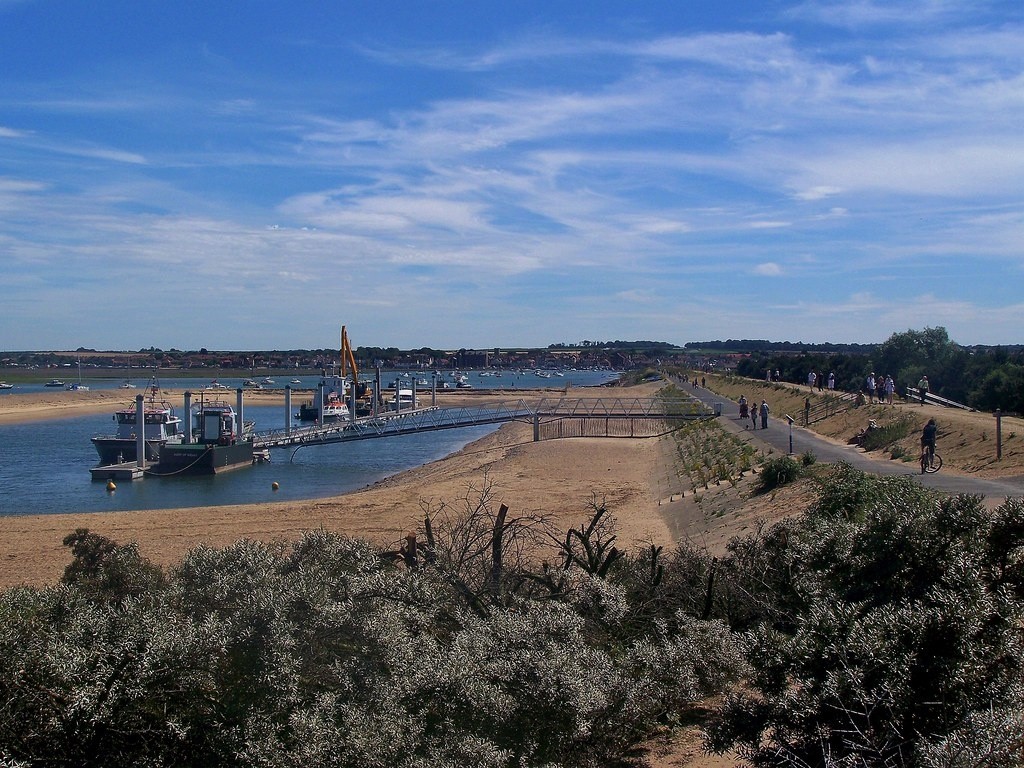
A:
[340,325,385,416]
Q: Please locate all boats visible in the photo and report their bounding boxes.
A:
[45,379,66,387]
[204,378,230,390]
[119,382,136,389]
[295,358,352,421]
[157,384,272,481]
[388,357,473,389]
[88,366,183,465]
[291,378,301,384]
[478,364,607,379]
[0,384,14,389]
[322,387,352,420]
[386,389,421,412]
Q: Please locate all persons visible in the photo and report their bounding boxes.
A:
[915,376,930,406]
[661,369,705,389]
[853,390,866,408]
[765,368,771,382]
[774,368,780,382]
[738,394,770,430]
[920,419,936,469]
[808,369,835,391]
[867,372,895,405]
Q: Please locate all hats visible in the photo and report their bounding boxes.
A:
[871,372,875,375]
[878,376,883,380]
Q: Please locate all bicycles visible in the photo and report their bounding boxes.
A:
[919,443,943,475]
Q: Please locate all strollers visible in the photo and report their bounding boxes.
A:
[739,403,750,420]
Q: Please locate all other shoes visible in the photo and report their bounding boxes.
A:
[930,466,936,469]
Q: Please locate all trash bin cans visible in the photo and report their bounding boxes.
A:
[714,402,724,415]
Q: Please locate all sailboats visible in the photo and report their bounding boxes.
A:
[66,360,90,391]
[242,359,275,387]
